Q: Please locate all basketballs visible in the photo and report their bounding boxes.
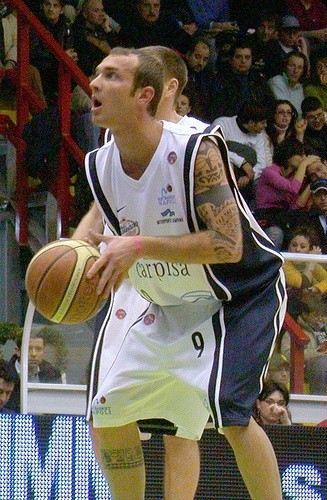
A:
[24,238,111,325]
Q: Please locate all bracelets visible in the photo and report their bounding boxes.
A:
[241,160,247,167]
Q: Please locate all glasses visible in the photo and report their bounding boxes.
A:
[276,110,295,116]
[265,397,290,409]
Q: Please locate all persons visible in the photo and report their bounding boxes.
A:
[0,0,327,425]
[69,47,288,500]
[84,46,242,500]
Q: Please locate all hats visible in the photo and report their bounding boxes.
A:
[310,179,327,195]
